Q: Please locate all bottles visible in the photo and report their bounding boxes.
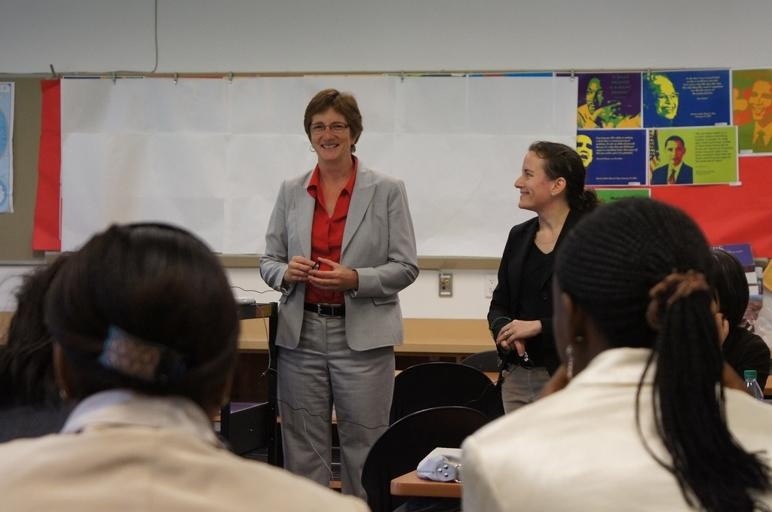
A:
[755,267,763,296]
[742,369,764,402]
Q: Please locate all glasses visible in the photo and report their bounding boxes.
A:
[308,121,350,132]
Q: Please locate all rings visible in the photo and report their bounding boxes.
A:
[504,330,509,339]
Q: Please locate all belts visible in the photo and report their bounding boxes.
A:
[301,300,347,318]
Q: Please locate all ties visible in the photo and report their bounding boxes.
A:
[668,168,676,185]
[754,129,766,149]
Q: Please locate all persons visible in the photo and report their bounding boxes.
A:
[734,75,772,152]
[488,141,607,414]
[454,197,772,512]
[703,245,772,400]
[649,132,694,186]
[267,89,422,501]
[0,220,379,511]
[573,134,594,168]
[0,254,75,447]
[580,76,628,129]
[645,72,687,128]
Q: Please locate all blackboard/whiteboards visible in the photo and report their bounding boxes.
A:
[59,69,579,270]
